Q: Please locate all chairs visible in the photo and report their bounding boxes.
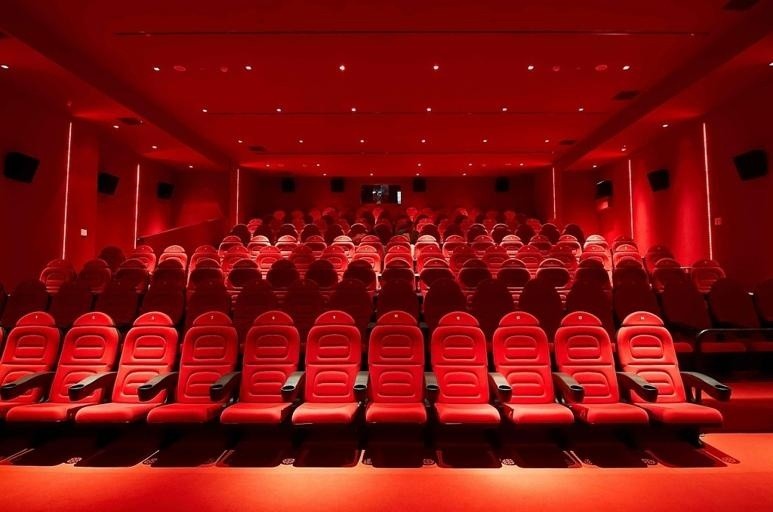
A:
[289,309,369,447]
[4,310,120,447]
[613,311,733,448]
[143,312,239,448]
[490,309,585,447]
[40,207,765,381]
[364,309,442,448]
[550,311,659,446]
[64,310,181,449]
[429,309,514,447]
[0,309,60,445]
[218,309,303,448]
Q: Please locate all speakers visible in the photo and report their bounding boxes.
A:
[331,179,344,192]
[3,151,40,183]
[98,173,118,194]
[283,179,295,192]
[596,181,612,197]
[733,149,768,180]
[649,169,669,191]
[413,179,425,192]
[496,177,510,192]
[158,183,173,198]
